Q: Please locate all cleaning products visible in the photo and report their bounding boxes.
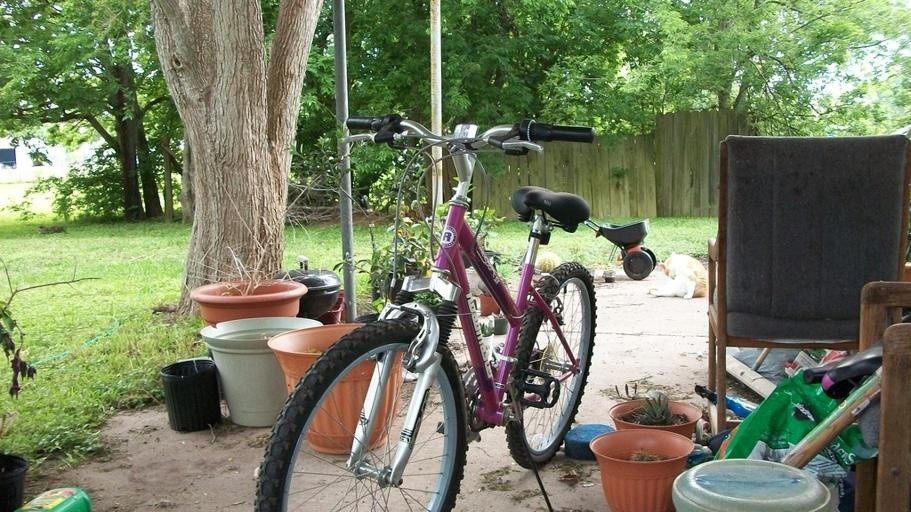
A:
[16,488,92,512]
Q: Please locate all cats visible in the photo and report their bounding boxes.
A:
[645,251,709,299]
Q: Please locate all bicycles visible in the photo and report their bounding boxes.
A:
[253,114,599,511]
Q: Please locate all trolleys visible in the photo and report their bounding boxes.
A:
[584,219,658,279]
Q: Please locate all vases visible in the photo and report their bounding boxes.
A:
[191,276,308,402]
[268,323,404,456]
[199,316,324,428]
[160,360,222,433]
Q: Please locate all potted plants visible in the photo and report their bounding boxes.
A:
[587,391,704,510]
